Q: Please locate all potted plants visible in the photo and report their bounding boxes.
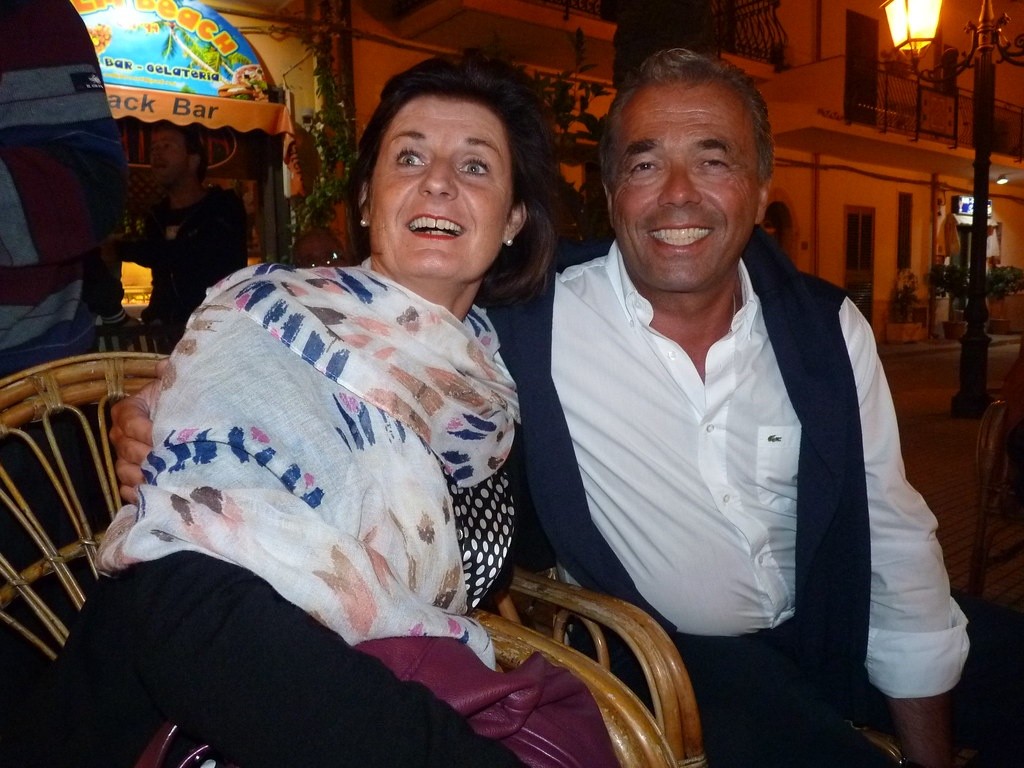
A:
[931,263,970,340]
[886,265,922,343]
[984,265,1024,335]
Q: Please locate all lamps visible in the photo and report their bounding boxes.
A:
[996,174,1008,185]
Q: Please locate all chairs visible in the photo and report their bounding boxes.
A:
[969,399,1024,602]
[0,347,976,768]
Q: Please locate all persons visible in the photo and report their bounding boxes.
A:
[487,49,1023,767]
[56,50,557,768]
[100,122,248,351]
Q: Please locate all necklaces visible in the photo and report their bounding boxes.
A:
[732,291,736,315]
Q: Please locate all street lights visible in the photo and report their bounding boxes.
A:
[879,0,1024,419]
